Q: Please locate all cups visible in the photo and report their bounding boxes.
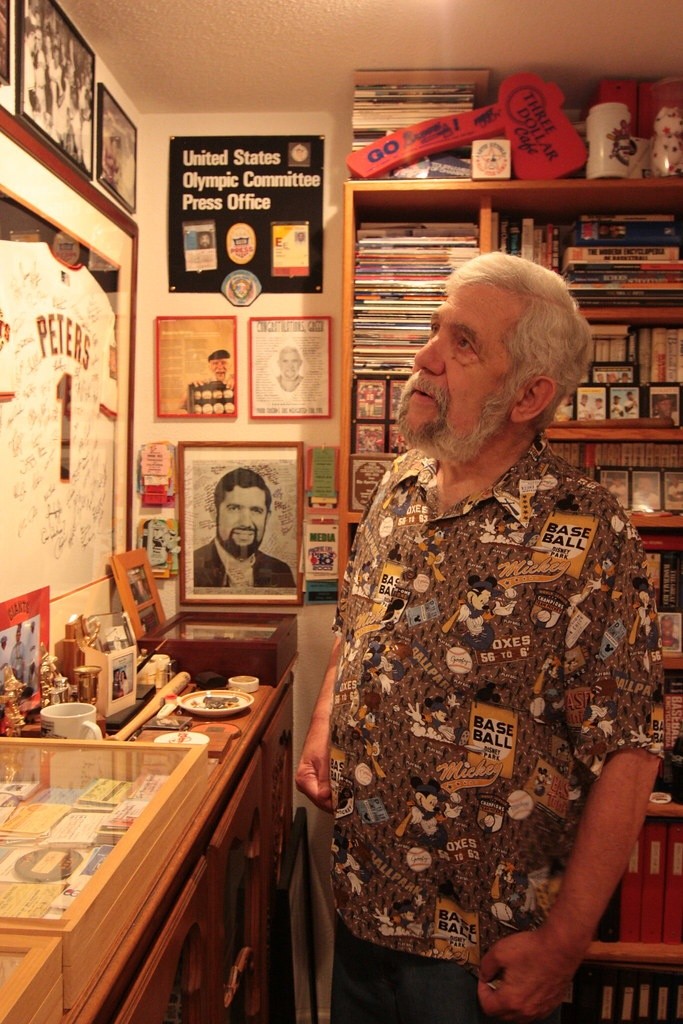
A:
[40,702,103,740]
[585,102,631,180]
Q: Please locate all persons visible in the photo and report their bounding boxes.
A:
[635,475,657,503]
[297,233,304,242]
[191,350,234,390]
[668,477,683,501]
[296,251,663,1024]
[197,232,213,249]
[661,616,677,647]
[276,347,304,392]
[608,473,625,494]
[595,372,631,383]
[611,392,638,417]
[656,395,678,424]
[0,621,37,686]
[577,395,605,420]
[113,669,130,698]
[361,384,383,415]
[25,27,92,167]
[193,469,295,588]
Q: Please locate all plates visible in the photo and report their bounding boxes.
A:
[153,730,210,745]
[178,690,255,718]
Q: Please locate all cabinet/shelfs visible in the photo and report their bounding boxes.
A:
[79,653,310,1024]
[337,177,683,819]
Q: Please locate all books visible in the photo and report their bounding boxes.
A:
[492,213,683,362]
[355,378,411,454]
[353,83,475,152]
[645,550,682,804]
[550,443,683,479]
[640,328,683,382]
[353,221,480,377]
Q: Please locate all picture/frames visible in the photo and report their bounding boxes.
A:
[250,317,331,419]
[180,438,305,606]
[17,0,97,178]
[156,314,238,419]
[96,82,138,215]
[0,0,11,86]
